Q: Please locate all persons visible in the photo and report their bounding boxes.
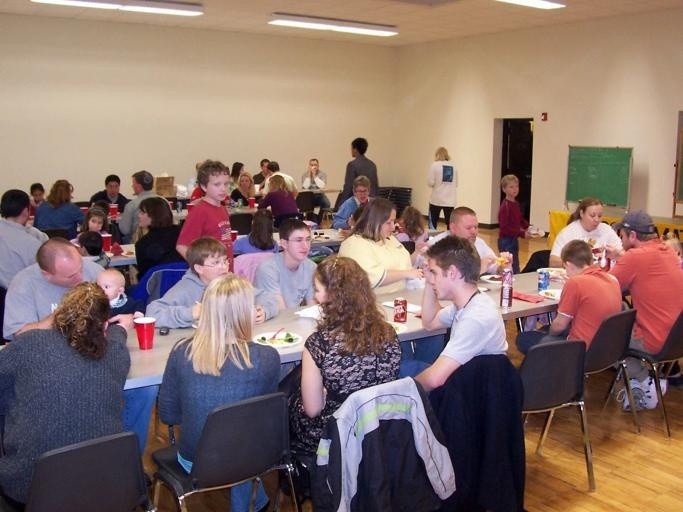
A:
[2,136,682,512]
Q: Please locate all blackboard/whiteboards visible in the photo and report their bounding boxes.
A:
[566,145,633,208]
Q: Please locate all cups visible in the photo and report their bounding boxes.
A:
[79,207,89,211]
[101,233,112,252]
[187,203,194,212]
[169,200,173,210]
[232,230,239,240]
[249,197,256,208]
[109,204,118,216]
[134,317,156,350]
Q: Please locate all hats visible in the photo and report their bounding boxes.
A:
[610,208,656,234]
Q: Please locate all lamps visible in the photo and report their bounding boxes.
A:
[30,0,205,17]
[266,13,398,37]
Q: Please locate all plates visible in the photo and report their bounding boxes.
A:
[480,275,514,282]
[254,332,301,347]
[539,288,563,301]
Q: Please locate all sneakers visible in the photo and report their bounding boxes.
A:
[616,378,644,413]
[643,377,668,410]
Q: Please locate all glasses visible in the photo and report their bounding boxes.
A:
[199,256,232,269]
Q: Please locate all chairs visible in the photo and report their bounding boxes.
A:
[150,392,302,512]
[520,250,551,272]
[0,285,11,345]
[24,430,153,512]
[439,353,524,512]
[273,377,426,512]
[321,192,342,227]
[41,229,68,238]
[296,192,314,216]
[518,340,595,493]
[584,308,641,434]
[601,309,683,436]
[401,240,415,255]
[230,213,253,235]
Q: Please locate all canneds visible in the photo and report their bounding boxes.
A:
[394,297,407,323]
[500,285,511,308]
[537,269,551,291]
[501,268,513,287]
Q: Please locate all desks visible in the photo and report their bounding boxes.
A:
[549,208,683,248]
